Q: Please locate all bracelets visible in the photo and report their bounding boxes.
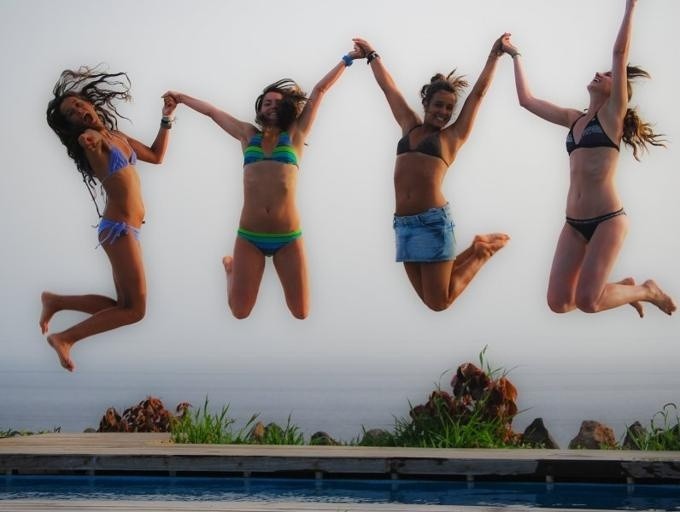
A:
[511,52,522,58]
[366,51,379,64]
[160,116,173,129]
[342,55,353,66]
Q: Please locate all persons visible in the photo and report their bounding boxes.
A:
[38,64,178,372]
[160,44,365,320]
[352,32,513,312]
[499,0,676,318]
